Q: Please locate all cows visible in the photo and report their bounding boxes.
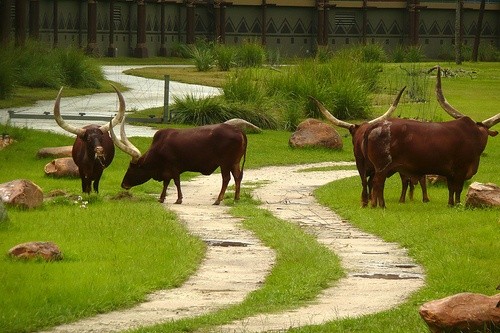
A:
[53,82,125,195]
[307,66,500,210]
[109,114,247,206]
